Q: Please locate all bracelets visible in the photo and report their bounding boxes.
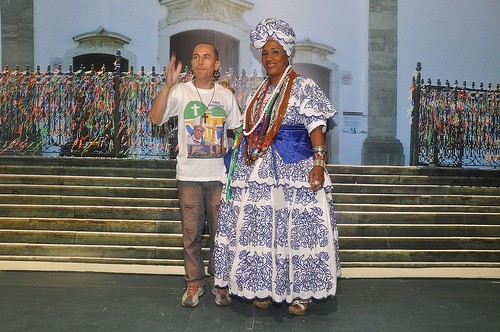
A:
[312,144,328,170]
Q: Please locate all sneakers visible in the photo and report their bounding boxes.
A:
[211,285,231,307]
[181,286,204,307]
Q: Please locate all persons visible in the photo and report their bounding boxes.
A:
[214,17,343,315]
[150,41,243,308]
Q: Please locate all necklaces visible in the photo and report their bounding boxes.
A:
[193,78,214,124]
[242,64,293,166]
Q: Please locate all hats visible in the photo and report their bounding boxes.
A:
[249,16,297,57]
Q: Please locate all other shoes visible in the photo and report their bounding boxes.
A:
[252,299,272,309]
[288,298,312,315]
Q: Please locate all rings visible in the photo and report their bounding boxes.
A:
[313,180,323,191]
